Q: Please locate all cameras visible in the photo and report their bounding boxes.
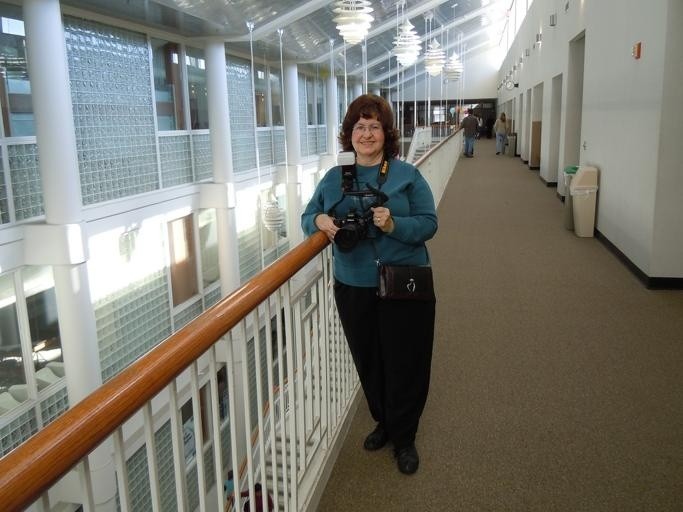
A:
[333,151,365,251]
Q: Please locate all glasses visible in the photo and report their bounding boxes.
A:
[353,122,382,132]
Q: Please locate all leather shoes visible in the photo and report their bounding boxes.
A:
[364,421,395,452]
[395,437,420,475]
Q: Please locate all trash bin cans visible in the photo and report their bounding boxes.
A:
[507,133,517,157]
[563,166,598,238]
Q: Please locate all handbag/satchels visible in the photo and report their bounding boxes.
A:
[355,168,436,303]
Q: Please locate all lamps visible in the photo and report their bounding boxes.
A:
[391,0,421,67]
[446,3,464,81]
[332,0,374,45]
[423,0,445,77]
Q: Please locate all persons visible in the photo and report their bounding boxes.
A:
[493,113,509,154]
[223,469,235,512]
[486,114,494,138]
[475,113,483,140]
[301,95,438,474]
[461,109,478,157]
[230,483,273,512]
